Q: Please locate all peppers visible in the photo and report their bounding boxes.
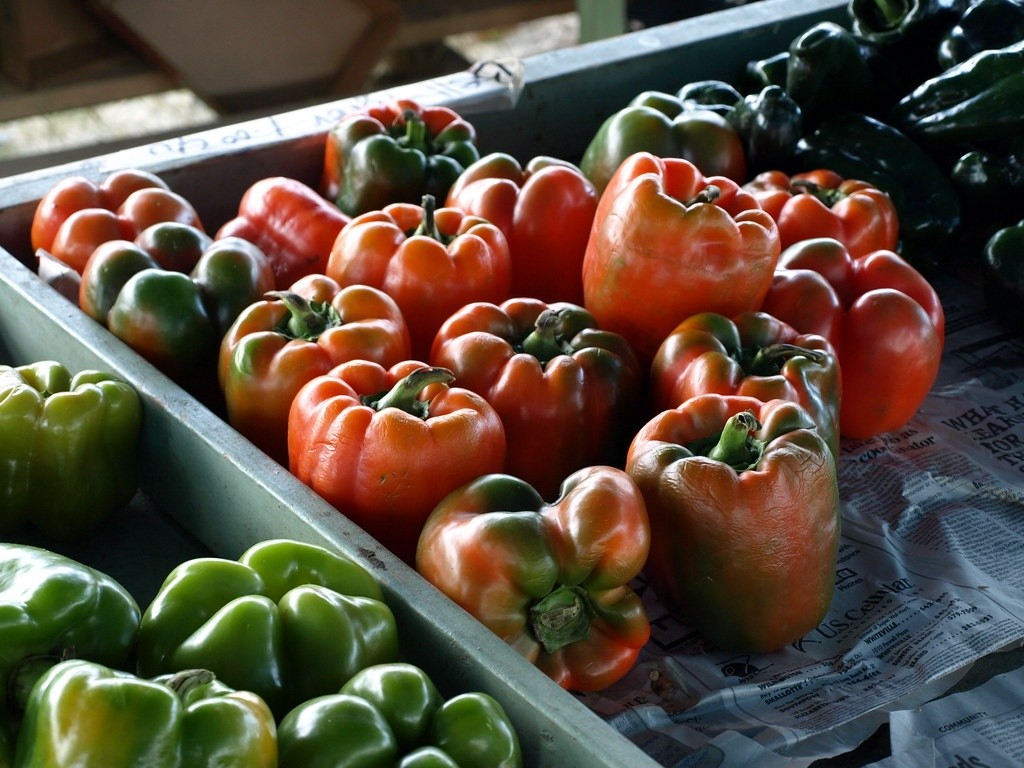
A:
[0,356,529,768]
[27,0,1024,690]
